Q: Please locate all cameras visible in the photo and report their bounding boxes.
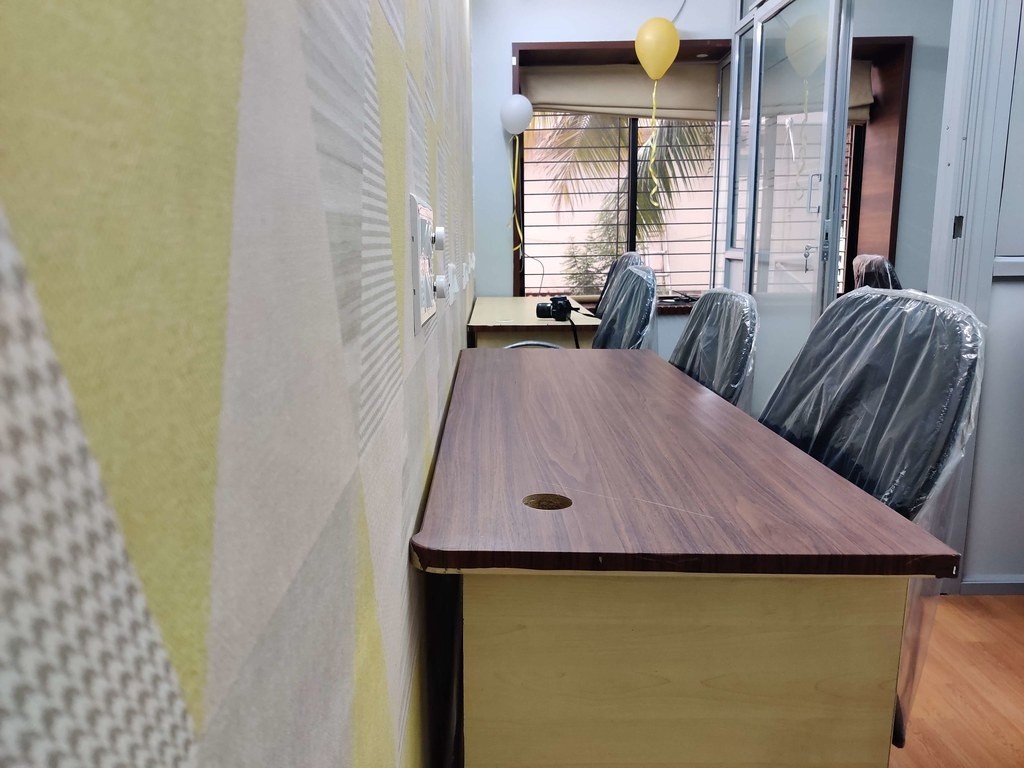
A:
[537,296,572,321]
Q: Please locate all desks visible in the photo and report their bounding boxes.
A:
[466,295,602,349]
[409,348,961,768]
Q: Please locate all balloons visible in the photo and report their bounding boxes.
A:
[500,94,533,134]
[785,15,827,77]
[634,17,680,81]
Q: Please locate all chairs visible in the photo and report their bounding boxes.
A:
[668,286,757,408]
[758,285,985,749]
[504,264,659,349]
[593,252,642,320]
[852,254,903,292]
